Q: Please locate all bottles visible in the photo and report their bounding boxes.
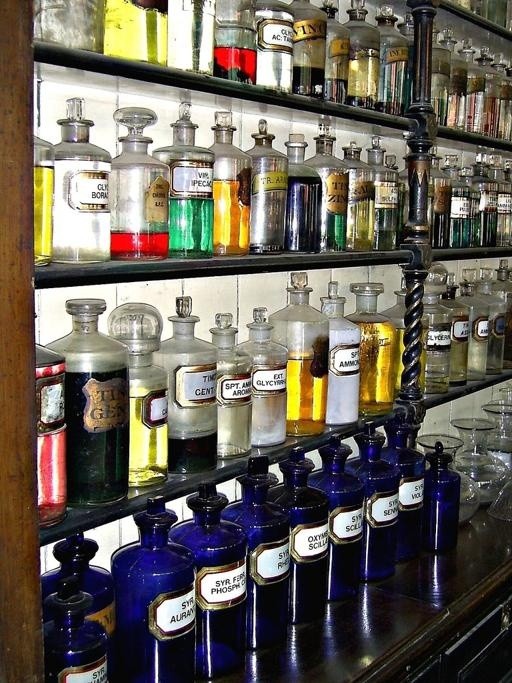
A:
[434,21,512,140]
[415,380,511,554]
[422,259,511,395]
[35,265,430,510]
[430,146,512,247]
[33,69,406,268]
[32,3,413,121]
[39,406,426,682]
[455,1,511,28]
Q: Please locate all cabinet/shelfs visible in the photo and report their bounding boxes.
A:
[1,1,512,683]
